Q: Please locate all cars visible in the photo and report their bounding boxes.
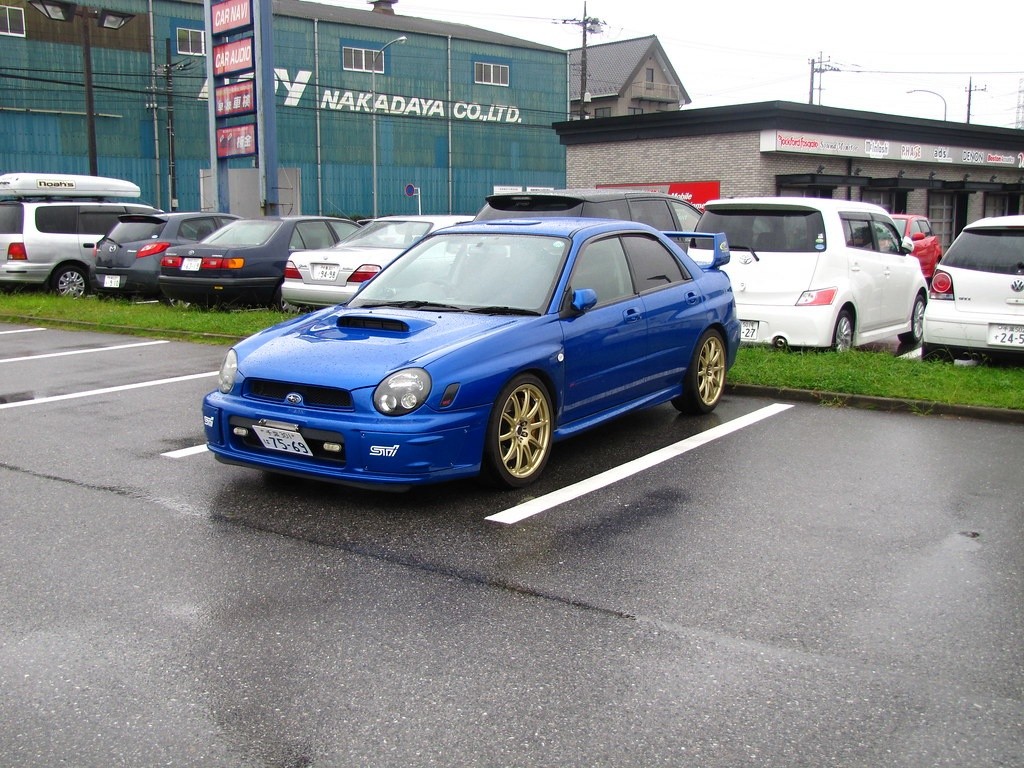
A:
[686,195,929,362]
[202,215,746,492]
[88,213,248,301]
[862,213,945,289]
[159,215,360,315]
[281,213,481,312]
[918,214,1023,369]
[470,189,708,275]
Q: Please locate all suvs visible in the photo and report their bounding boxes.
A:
[0,172,197,302]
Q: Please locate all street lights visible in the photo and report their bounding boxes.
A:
[906,88,947,123]
[371,36,408,216]
